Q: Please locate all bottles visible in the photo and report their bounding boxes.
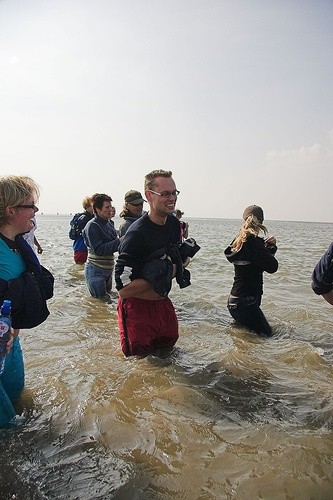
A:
[0,300,12,375]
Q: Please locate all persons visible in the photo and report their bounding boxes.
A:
[224,204,278,337]
[117,169,191,358]
[114,189,148,292]
[312,242,333,307]
[0,176,43,430]
[73,193,124,299]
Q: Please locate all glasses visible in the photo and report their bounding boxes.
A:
[16,204,35,208]
[148,189,180,197]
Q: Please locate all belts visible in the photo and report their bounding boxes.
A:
[228,298,255,304]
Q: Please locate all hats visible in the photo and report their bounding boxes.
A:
[124,190,147,205]
[242,204,264,223]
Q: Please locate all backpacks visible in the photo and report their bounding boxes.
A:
[69,213,88,240]
